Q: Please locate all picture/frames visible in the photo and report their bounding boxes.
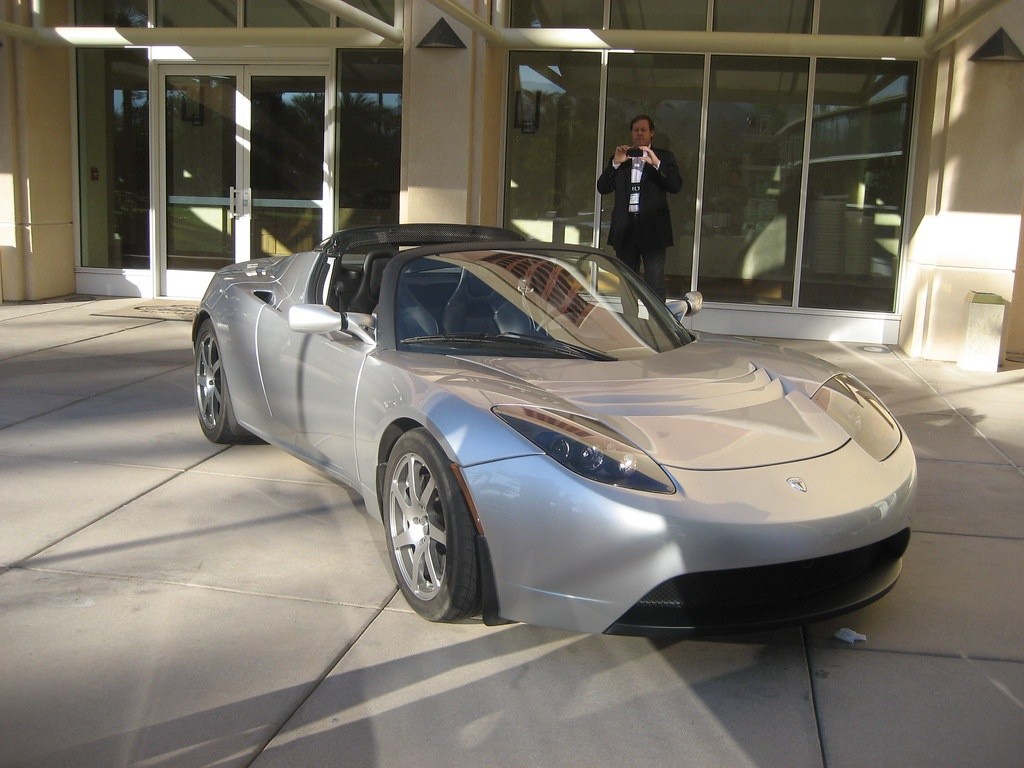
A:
[514,89,540,129]
[182,85,205,122]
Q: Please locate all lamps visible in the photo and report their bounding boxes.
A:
[967,26,1024,63]
[416,16,467,49]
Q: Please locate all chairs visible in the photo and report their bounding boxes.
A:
[441,266,536,336]
[347,249,438,339]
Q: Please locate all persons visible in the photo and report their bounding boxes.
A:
[596,114,683,322]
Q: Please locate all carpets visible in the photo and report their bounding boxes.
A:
[90,298,199,322]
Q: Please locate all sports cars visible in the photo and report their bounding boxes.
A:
[191,222,919,624]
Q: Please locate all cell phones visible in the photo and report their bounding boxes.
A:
[626,148,643,157]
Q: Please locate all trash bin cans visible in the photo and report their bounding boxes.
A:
[956,289,1006,374]
